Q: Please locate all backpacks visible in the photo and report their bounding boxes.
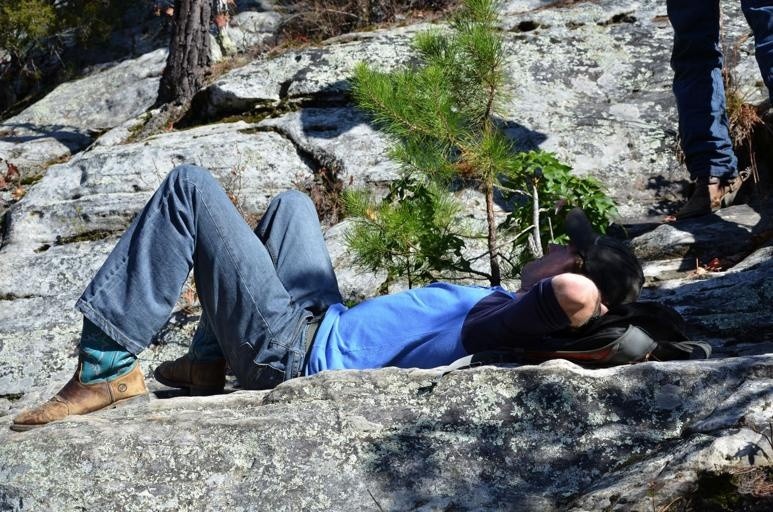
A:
[555,302,712,361]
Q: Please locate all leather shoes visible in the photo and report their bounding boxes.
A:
[677,174,743,220]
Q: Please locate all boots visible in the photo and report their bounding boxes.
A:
[11,316,148,431]
[154,311,225,395]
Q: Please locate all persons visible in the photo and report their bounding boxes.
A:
[10,164,645,432]
[667,0,773,219]
[154,0,176,17]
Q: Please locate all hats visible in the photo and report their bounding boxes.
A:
[562,206,645,306]
